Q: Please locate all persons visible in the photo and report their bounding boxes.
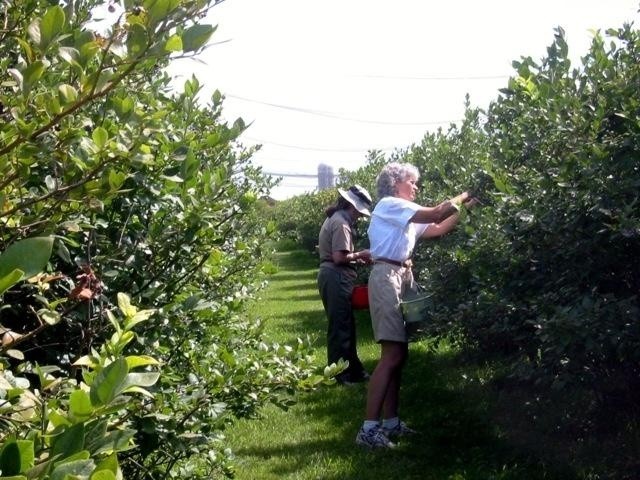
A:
[315,184,373,387]
[354,160,480,450]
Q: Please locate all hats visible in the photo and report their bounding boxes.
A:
[337,184,373,217]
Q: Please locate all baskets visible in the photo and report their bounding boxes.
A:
[400,284,437,323]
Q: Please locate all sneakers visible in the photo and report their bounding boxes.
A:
[356,423,398,453]
[337,370,371,386]
[381,420,423,439]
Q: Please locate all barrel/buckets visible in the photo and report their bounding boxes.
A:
[400,295,429,323]
[350,284,369,309]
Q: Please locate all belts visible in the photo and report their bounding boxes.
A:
[377,258,407,268]
